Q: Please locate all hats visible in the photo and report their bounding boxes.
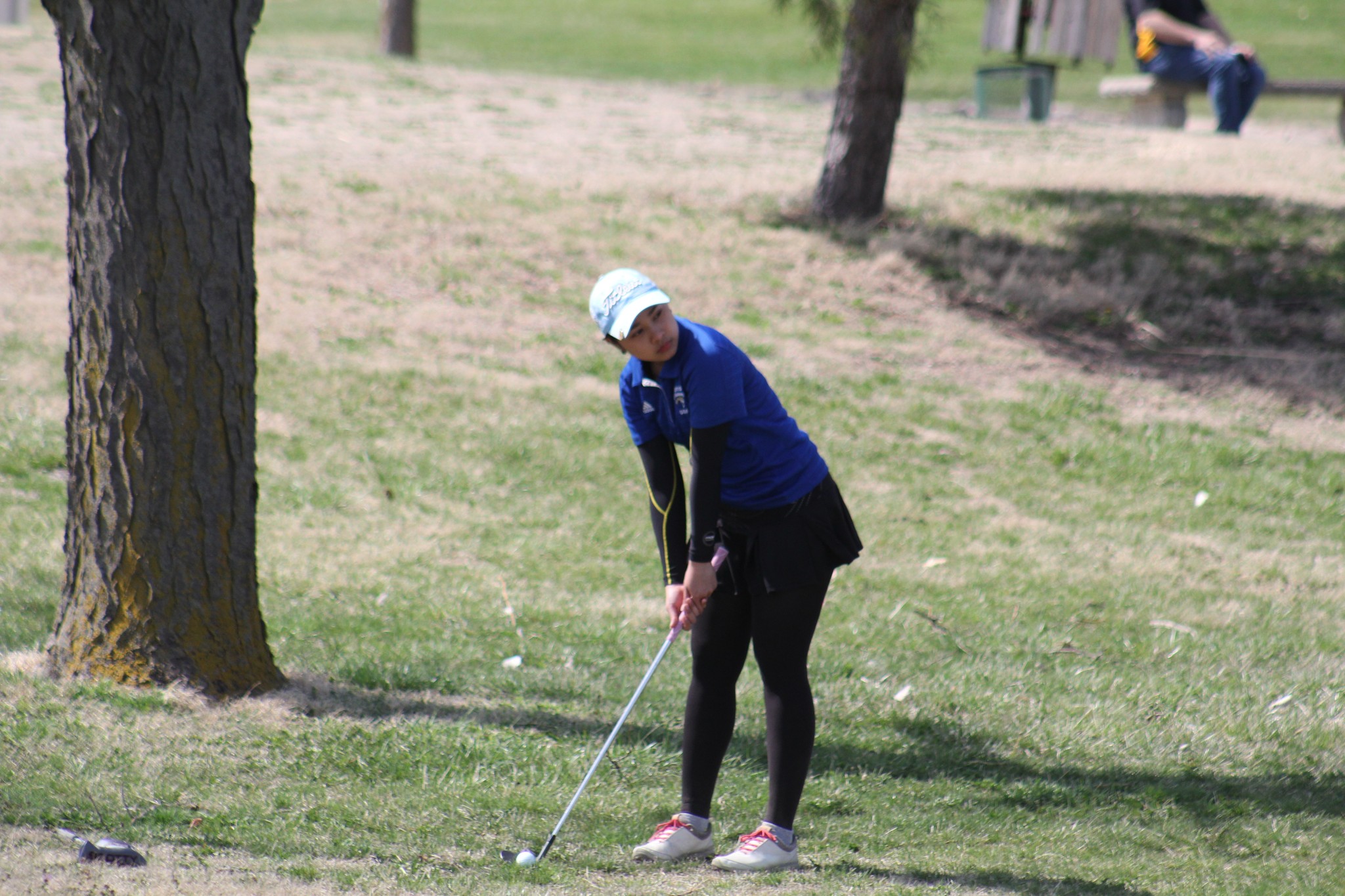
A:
[588,268,671,341]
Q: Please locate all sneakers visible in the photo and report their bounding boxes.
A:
[632,814,714,865]
[711,824,799,872]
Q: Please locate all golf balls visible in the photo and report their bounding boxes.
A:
[515,851,536,867]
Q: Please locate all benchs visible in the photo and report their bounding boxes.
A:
[1097,73,1345,130]
[976,61,1059,121]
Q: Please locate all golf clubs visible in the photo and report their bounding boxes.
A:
[499,607,683,865]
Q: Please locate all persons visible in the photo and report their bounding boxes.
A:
[1121,0,1267,135]
[589,269,863,873]
